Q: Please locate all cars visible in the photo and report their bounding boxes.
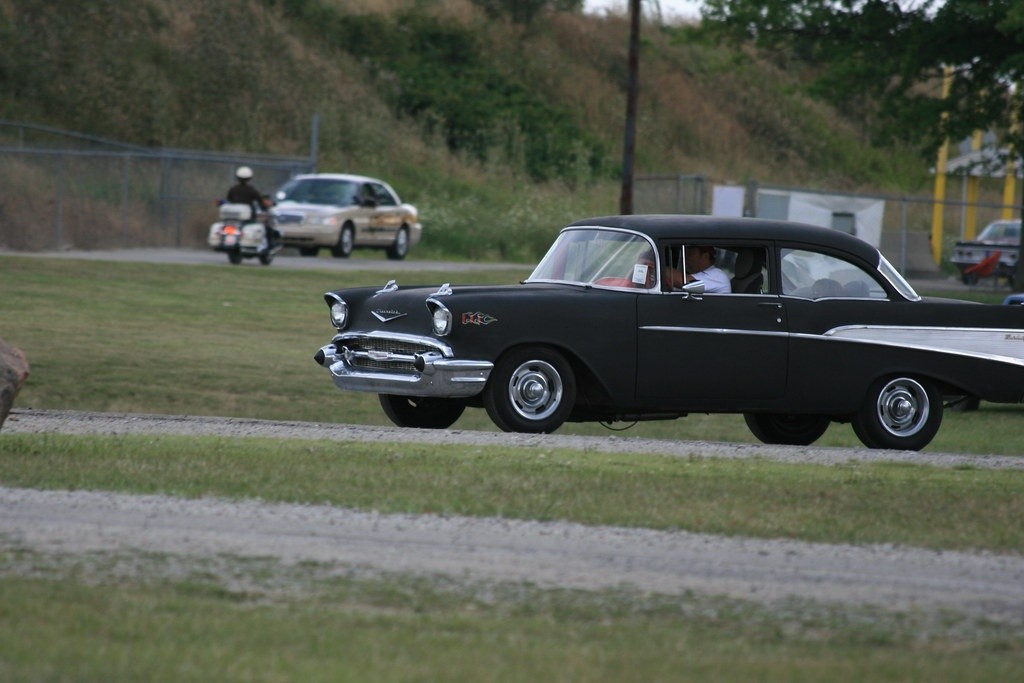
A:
[264,172,423,261]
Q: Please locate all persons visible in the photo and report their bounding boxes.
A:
[641,246,731,294]
[811,278,869,299]
[226,167,273,250]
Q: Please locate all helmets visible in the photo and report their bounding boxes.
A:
[237,167,253,180]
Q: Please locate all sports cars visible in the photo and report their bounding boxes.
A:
[950,218,1023,294]
[313,209,1024,453]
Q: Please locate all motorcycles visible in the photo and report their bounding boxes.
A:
[208,194,285,265]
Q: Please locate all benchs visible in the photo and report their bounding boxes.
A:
[786,281,870,298]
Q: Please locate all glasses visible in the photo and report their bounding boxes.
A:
[810,290,820,295]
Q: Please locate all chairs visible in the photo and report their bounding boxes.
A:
[649,247,681,292]
[730,249,763,294]
